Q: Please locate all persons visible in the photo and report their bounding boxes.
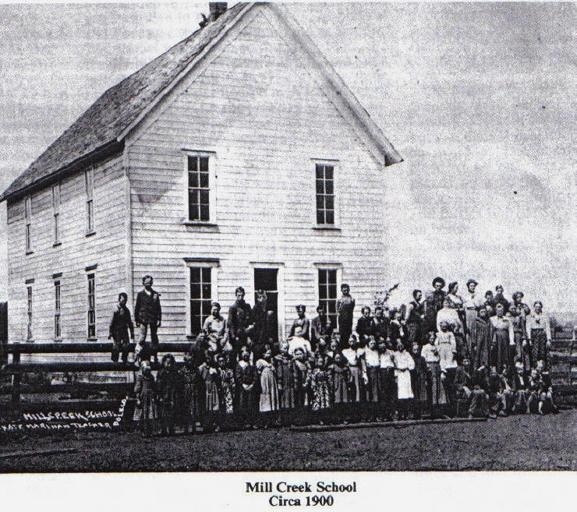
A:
[185,276,560,418]
[106,291,134,368]
[132,275,162,357]
[131,361,162,442]
[154,353,185,436]
